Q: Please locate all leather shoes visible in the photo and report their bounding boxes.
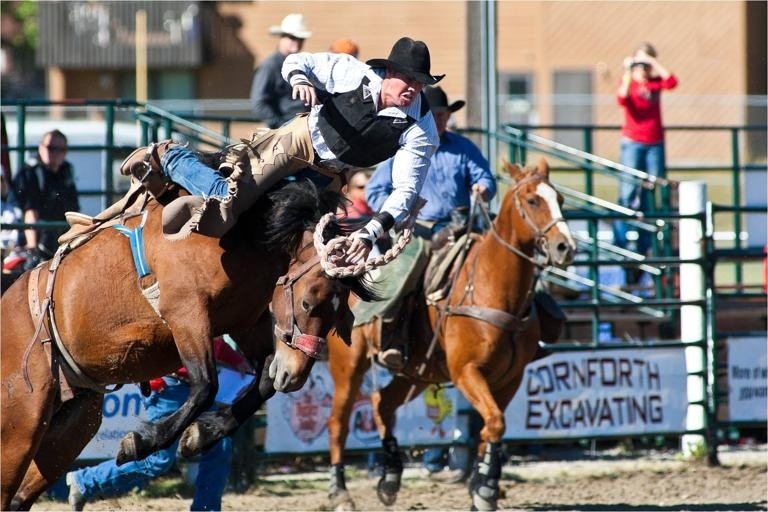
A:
[120,142,176,175]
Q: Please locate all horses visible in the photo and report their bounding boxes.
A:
[324,155,579,511]
[0,159,393,512]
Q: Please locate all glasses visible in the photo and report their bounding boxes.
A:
[42,143,68,152]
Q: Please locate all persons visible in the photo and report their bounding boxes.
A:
[13,128,84,269]
[333,163,379,222]
[361,102,499,369]
[245,13,314,134]
[58,333,254,511]
[612,41,677,253]
[117,35,449,268]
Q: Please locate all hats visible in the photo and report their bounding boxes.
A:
[269,14,312,39]
[330,39,358,54]
[365,37,464,112]
